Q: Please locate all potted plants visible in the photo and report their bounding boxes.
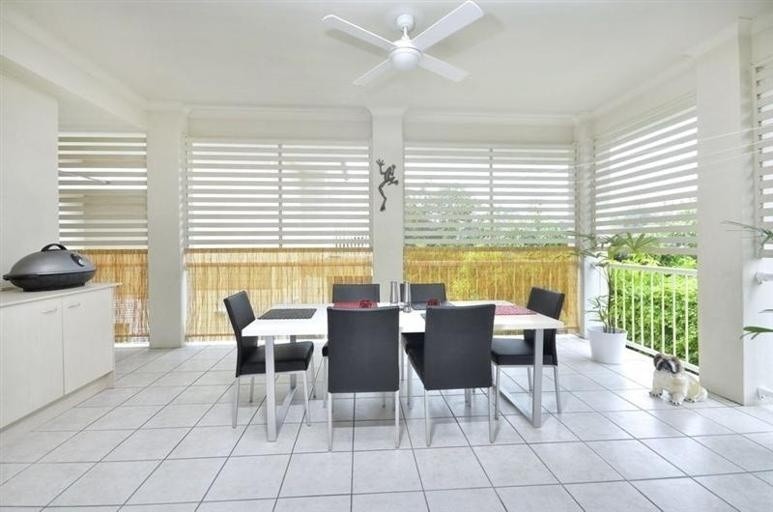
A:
[554,221,669,365]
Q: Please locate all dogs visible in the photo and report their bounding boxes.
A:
[649,352,708,406]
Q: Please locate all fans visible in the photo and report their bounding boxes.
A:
[320,0,483,88]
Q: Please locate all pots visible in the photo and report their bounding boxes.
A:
[3,243,97,291]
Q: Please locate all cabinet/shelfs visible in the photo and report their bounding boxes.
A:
[0,286,118,431]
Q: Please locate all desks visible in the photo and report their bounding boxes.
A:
[240,299,564,443]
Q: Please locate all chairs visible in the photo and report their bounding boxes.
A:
[490,285,565,419]
[398,282,449,382]
[321,283,382,406]
[325,304,403,452]
[408,300,497,449]
[223,288,316,430]
